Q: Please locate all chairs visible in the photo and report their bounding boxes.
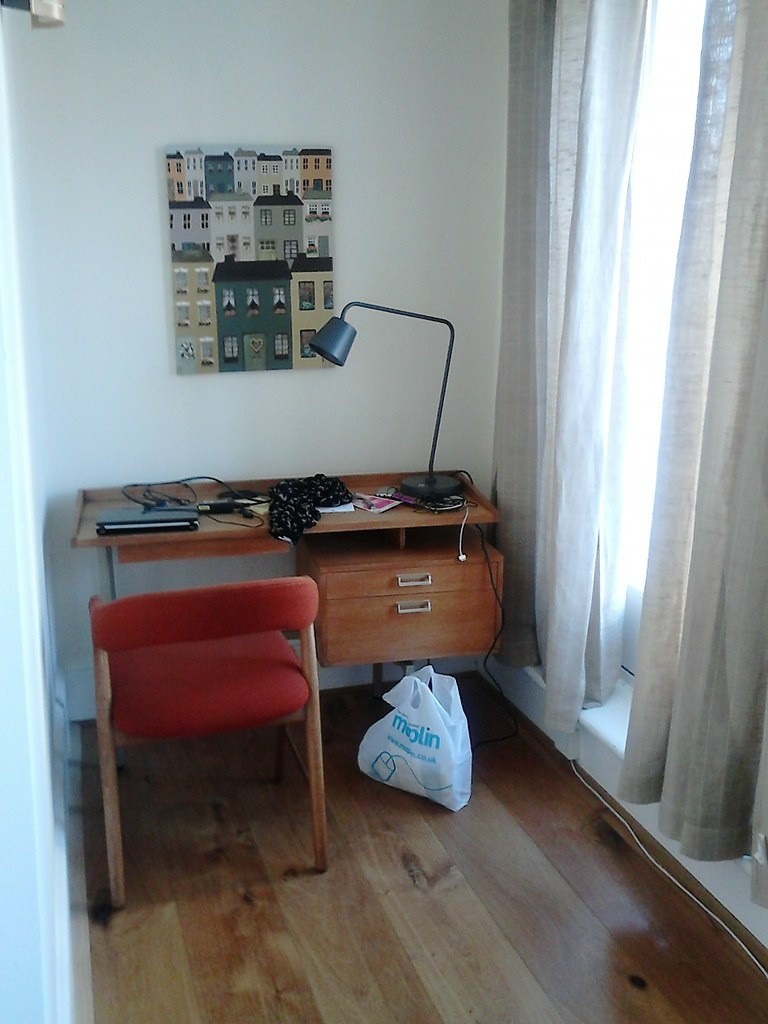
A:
[88,576,329,909]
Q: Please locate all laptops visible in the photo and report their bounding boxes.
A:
[95,504,199,536]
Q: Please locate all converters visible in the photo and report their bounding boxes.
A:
[198,498,235,515]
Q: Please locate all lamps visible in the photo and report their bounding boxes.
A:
[307,302,475,502]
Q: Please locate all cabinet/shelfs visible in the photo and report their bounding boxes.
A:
[294,524,504,667]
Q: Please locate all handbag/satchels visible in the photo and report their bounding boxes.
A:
[357,665,472,812]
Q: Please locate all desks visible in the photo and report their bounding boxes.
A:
[69,470,500,601]
